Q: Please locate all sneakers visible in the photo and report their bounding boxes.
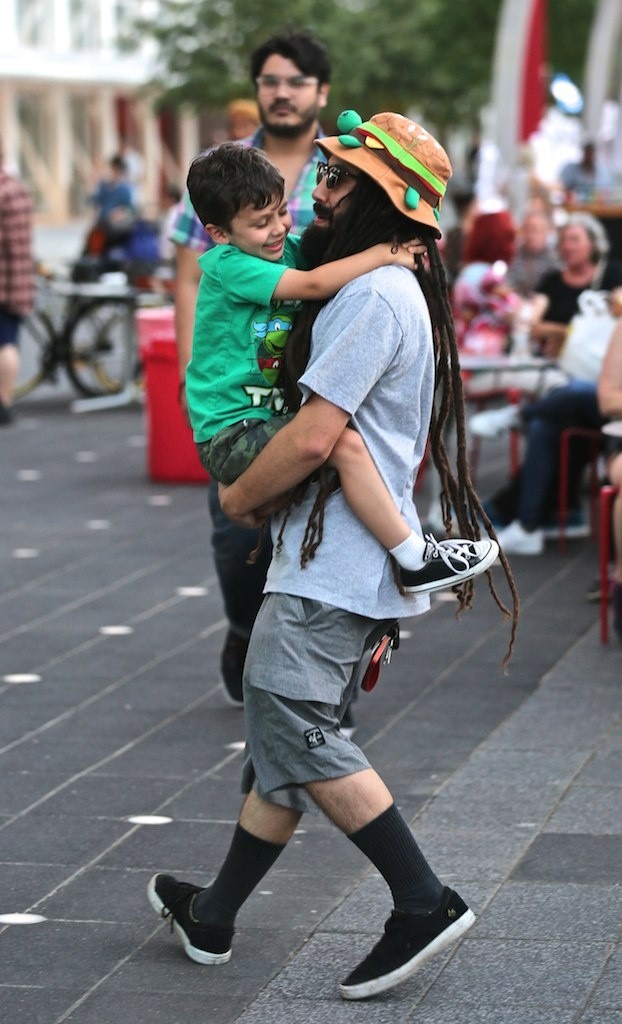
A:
[338,885,477,1001]
[445,495,506,534]
[398,537,499,595]
[481,517,543,555]
[543,510,591,540]
[146,872,236,965]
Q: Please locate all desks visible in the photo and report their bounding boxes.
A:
[414,352,558,535]
[45,280,164,393]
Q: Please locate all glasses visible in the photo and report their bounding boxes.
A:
[254,74,321,87]
[315,160,359,188]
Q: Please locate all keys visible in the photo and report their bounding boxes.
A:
[370,621,402,668]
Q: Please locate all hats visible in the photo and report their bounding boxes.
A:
[312,108,453,240]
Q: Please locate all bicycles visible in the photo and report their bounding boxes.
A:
[9,265,147,401]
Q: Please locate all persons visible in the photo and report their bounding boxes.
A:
[76,93,264,299]
[168,30,358,746]
[187,143,501,597]
[468,372,604,556]
[0,148,37,428]
[595,310,622,592]
[447,173,622,370]
[148,112,523,1000]
